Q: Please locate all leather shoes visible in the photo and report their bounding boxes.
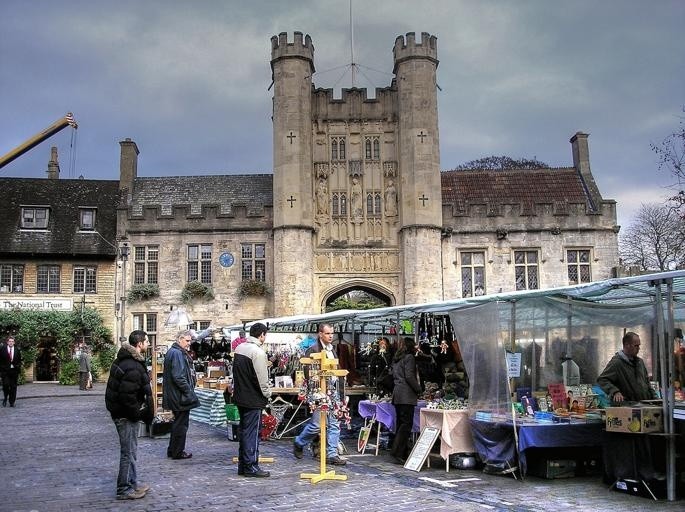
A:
[166,449,193,460]
[133,484,151,493]
[115,488,147,501]
[237,466,271,477]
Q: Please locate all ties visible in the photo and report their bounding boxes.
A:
[9,347,13,360]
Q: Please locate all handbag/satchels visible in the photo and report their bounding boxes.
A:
[223,392,240,421]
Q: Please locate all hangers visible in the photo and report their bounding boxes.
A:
[265,394,294,409]
[262,409,269,417]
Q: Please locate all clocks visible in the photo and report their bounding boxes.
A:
[219,252,234,267]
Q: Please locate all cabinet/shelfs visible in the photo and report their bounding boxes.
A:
[150,350,172,418]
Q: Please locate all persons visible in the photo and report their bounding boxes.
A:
[234,323,271,477]
[571,400,579,412]
[293,322,346,465]
[86,348,93,388]
[665,327,685,388]
[595,330,655,495]
[367,336,447,462]
[567,390,574,404]
[1,337,21,407]
[105,330,155,499]
[79,348,91,390]
[162,331,201,459]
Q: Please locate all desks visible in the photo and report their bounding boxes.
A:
[189,386,231,428]
[468,412,605,481]
[357,399,432,457]
[418,407,477,473]
[269,387,374,439]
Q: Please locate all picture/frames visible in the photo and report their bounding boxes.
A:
[403,425,442,472]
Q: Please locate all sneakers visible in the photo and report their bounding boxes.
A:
[326,454,347,466]
[1,400,7,408]
[390,454,406,464]
[9,401,16,408]
[293,437,303,460]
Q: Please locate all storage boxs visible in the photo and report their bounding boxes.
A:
[545,459,576,479]
[197,370,230,390]
[223,404,242,420]
[606,405,663,434]
